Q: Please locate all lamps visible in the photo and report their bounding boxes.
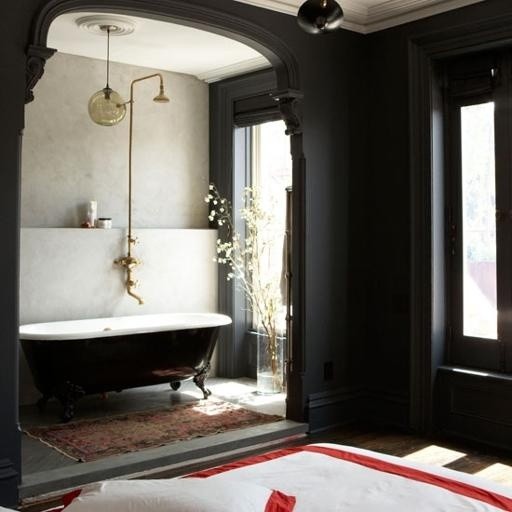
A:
[88,25,126,126]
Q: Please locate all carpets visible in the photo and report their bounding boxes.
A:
[23,398,285,463]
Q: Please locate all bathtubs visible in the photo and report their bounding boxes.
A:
[18,311,233,423]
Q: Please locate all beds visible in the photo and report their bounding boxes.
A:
[41,441,512,512]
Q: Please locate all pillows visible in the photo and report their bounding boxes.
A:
[61,481,296,512]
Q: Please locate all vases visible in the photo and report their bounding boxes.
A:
[257,325,286,395]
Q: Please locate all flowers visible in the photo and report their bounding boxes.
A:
[204,183,284,392]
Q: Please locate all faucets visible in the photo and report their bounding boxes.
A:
[125,278,145,305]
